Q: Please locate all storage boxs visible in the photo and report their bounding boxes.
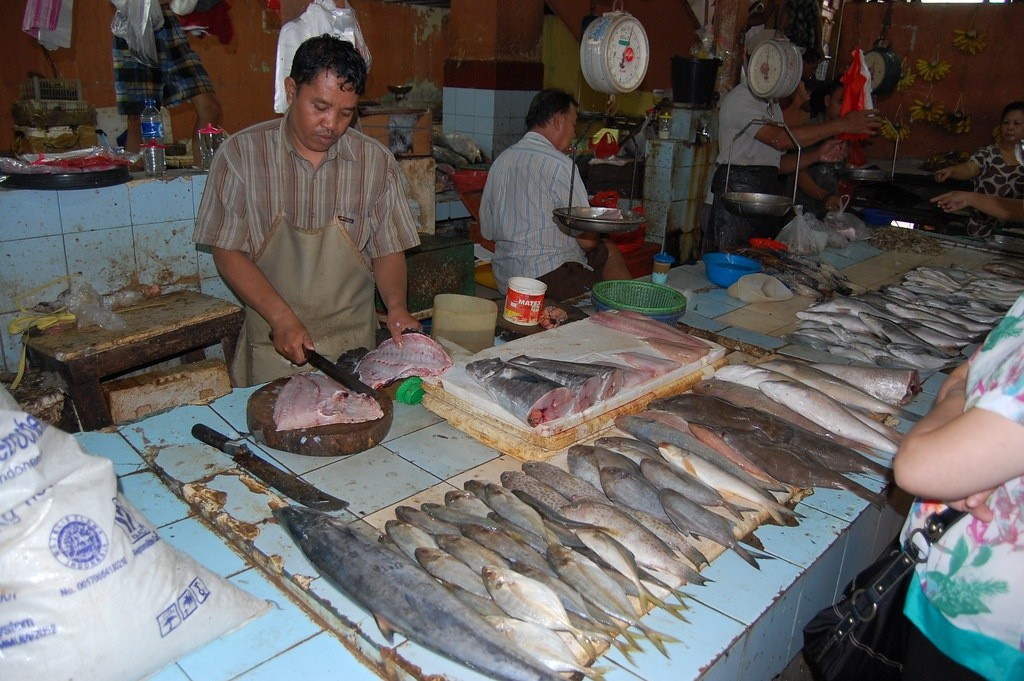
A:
[101,357,232,426]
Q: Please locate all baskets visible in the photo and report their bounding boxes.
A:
[590,280,687,327]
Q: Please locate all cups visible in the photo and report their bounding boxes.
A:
[654,90,664,105]
[652,260,671,284]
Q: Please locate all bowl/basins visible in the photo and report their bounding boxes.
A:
[702,253,762,288]
[452,170,487,193]
[863,209,897,227]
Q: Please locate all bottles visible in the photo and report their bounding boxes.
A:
[337,347,426,405]
[659,113,671,139]
[198,123,224,172]
[141,101,166,177]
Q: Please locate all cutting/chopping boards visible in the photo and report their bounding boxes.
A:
[247,374,394,457]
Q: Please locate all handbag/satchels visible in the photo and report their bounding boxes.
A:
[802,544,953,681]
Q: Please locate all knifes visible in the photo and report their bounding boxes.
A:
[268,331,380,400]
[191,424,350,511]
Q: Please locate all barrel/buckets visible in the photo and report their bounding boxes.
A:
[432,294,498,356]
[671,55,722,105]
[503,277,547,326]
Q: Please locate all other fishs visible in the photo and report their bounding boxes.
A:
[281,312,927,681]
[269,504,569,681]
[737,246,854,303]
[779,262,1024,378]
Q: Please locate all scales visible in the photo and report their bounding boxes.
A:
[719,0,804,219]
[835,0,899,184]
[552,0,650,234]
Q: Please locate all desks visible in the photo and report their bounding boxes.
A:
[21,290,246,433]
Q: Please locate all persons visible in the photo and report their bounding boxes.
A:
[478,87,631,301]
[891,294,1024,681]
[700,29,883,254]
[112,0,220,163]
[192,34,423,388]
[929,100,1024,238]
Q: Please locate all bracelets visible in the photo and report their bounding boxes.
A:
[822,193,835,203]
